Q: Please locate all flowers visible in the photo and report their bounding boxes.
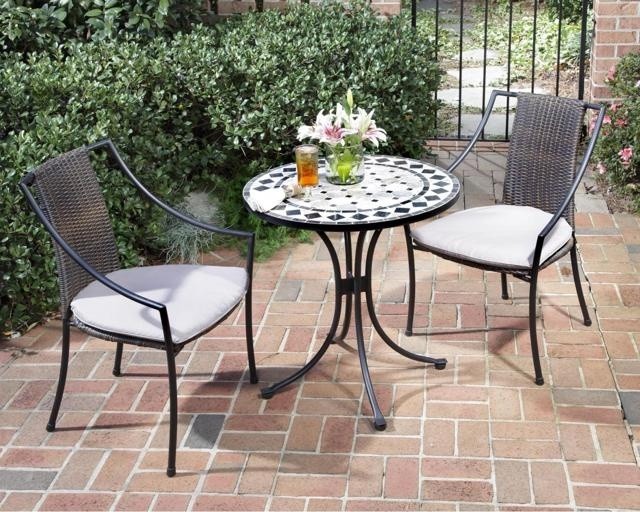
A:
[298,85,389,170]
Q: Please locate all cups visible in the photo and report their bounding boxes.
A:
[296,144,319,186]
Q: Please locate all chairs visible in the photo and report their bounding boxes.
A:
[18,137,260,481]
[401,87,608,388]
